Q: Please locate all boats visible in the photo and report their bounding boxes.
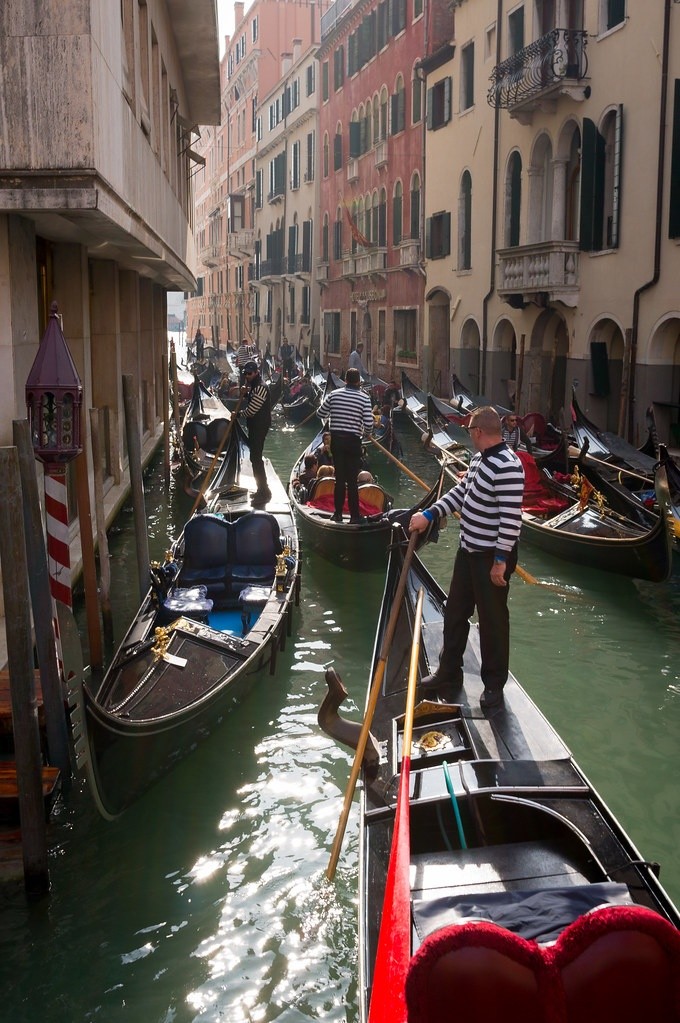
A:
[316,521,680,1023]
[288,417,449,573]
[186,337,679,584]
[78,416,302,756]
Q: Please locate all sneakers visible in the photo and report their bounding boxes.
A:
[480,684,502,706]
[421,672,465,690]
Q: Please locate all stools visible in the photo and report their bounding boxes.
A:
[240,586,273,634]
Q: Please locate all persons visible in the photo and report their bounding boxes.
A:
[192,329,204,363]
[348,342,373,376]
[236,340,255,386]
[213,371,237,395]
[315,369,374,524]
[362,380,400,441]
[230,362,271,503]
[502,412,532,455]
[299,432,373,492]
[408,406,524,705]
[278,337,293,382]
[289,377,315,403]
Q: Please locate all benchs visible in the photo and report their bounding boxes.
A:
[179,511,280,599]
[515,451,570,517]
[183,418,231,450]
[500,412,556,452]
[311,477,387,513]
[406,905,680,1023]
[447,413,473,426]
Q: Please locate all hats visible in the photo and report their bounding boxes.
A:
[244,362,257,370]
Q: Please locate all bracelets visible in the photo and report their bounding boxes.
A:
[494,560,504,564]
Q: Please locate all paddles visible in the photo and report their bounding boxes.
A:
[370,436,540,585]
[190,381,249,520]
[568,444,655,484]
[265,350,293,382]
[295,410,317,430]
[430,439,469,469]
[242,320,254,343]
[323,508,423,880]
[365,586,425,1022]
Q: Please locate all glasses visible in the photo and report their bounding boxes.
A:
[511,419,518,422]
[244,371,252,375]
[465,426,482,434]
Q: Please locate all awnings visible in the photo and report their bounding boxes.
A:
[186,148,206,179]
[177,115,201,156]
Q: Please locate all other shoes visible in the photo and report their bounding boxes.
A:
[250,489,272,505]
[330,513,342,520]
[350,515,366,524]
